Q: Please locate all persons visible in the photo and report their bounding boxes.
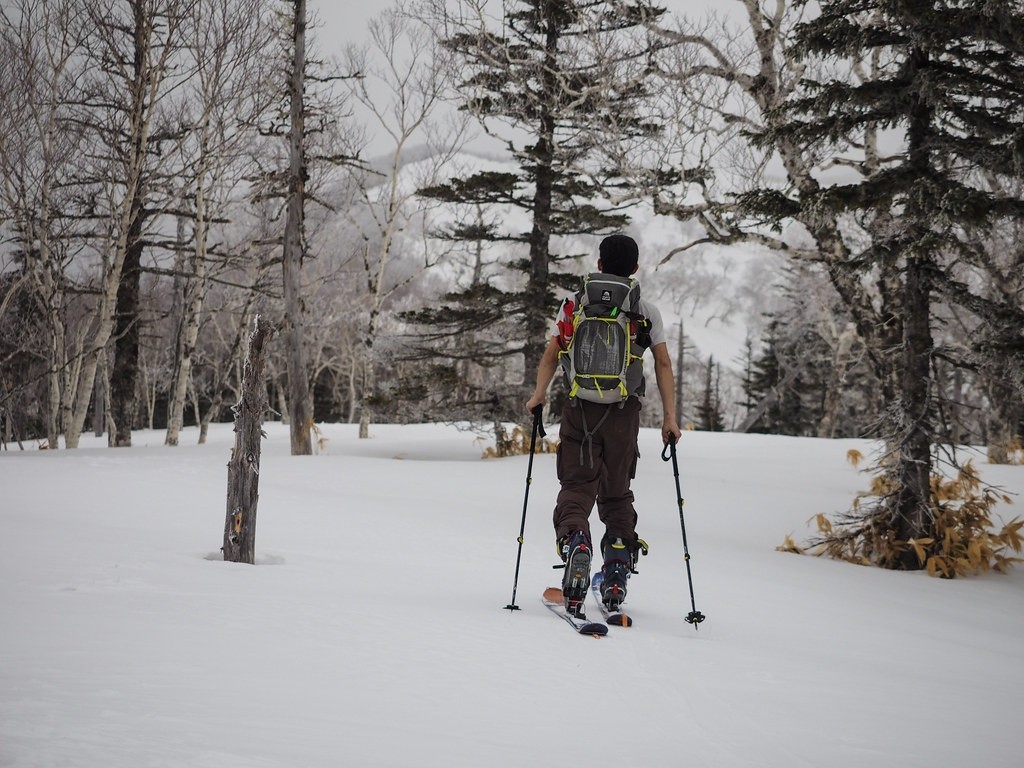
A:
[527,235,683,612]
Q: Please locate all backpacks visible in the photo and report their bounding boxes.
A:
[558,272,653,409]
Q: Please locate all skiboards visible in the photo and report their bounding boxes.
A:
[541,571,634,642]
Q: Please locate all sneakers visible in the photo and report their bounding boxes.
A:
[599,537,649,604]
[553,530,593,615]
